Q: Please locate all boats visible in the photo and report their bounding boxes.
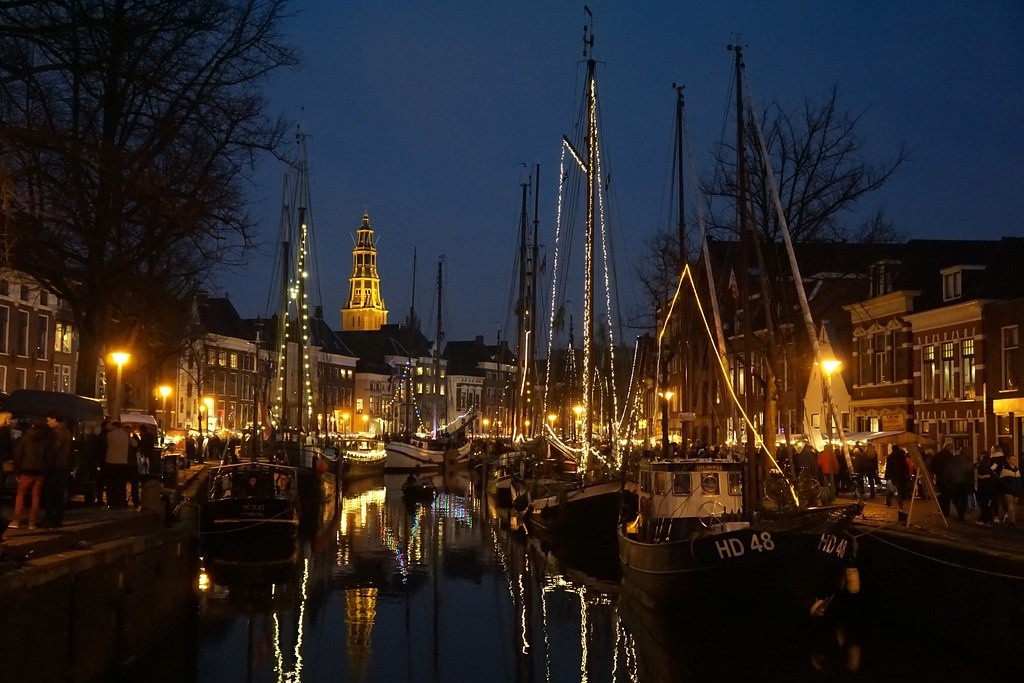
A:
[401,471,439,494]
[340,439,387,477]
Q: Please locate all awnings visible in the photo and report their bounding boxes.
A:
[825,431,940,445]
[0,390,105,423]
[120,412,157,427]
[734,433,803,447]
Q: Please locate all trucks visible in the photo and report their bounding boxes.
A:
[8,388,106,508]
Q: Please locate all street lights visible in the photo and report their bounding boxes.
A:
[109,350,131,419]
[157,385,171,435]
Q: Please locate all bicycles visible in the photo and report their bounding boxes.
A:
[764,459,821,502]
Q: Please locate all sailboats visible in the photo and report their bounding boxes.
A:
[477,7,623,533]
[208,105,343,533]
[617,33,865,594]
[385,246,474,468]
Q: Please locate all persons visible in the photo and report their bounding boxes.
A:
[164,433,241,464]
[0,411,155,530]
[883,442,1022,530]
[649,439,879,500]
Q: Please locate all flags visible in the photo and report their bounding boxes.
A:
[605,172,611,190]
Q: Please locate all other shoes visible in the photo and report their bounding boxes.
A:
[899,500,904,509]
[885,501,891,506]
[835,484,857,495]
[966,513,1011,530]
